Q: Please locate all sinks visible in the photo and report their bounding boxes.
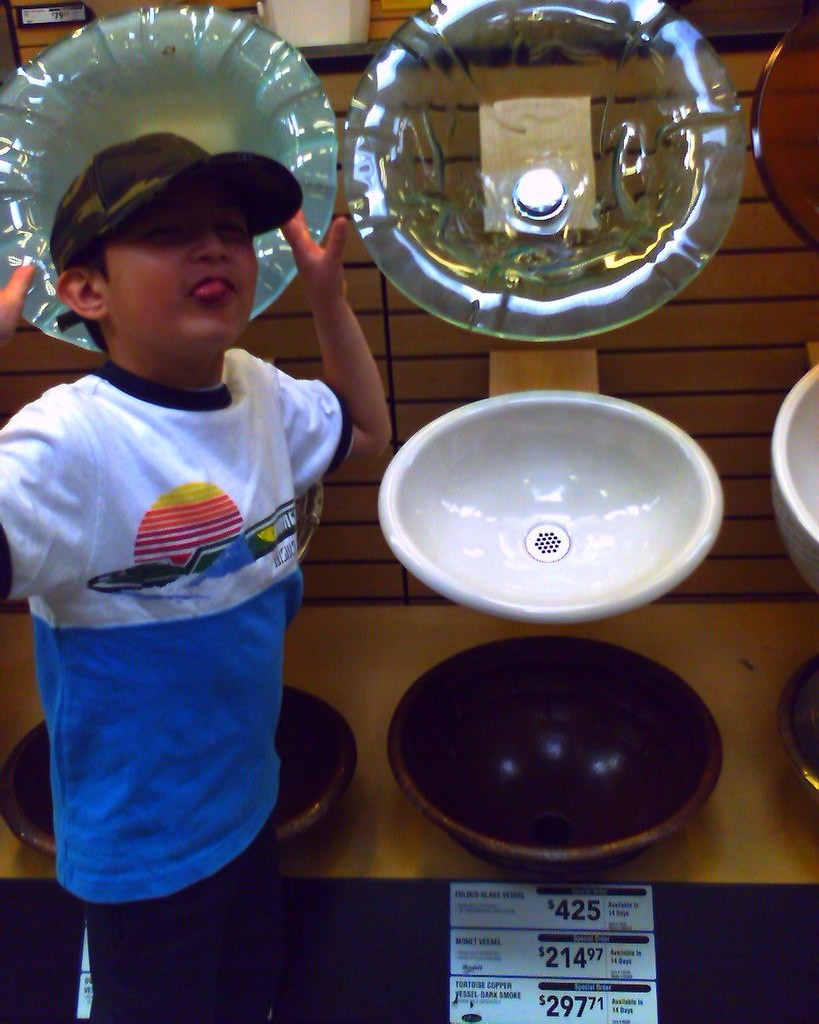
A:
[403,632,722,872]
[1,690,354,856]
[341,0,745,344]
[753,15,819,242]
[0,5,338,354]
[776,654,819,790]
[768,364,819,589]
[377,388,725,627]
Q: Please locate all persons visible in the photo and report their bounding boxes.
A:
[0,133,392,1024]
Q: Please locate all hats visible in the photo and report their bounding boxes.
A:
[50,132,302,274]
